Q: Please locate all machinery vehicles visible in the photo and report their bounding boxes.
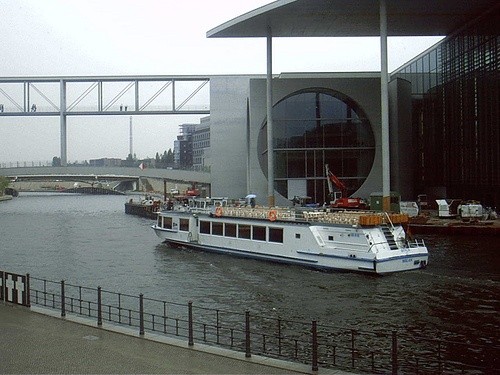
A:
[325,168,370,211]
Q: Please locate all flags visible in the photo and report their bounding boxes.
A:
[328,171,346,188]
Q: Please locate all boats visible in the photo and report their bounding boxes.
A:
[151,191,431,276]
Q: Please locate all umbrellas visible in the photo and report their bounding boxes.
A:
[245,194,256,199]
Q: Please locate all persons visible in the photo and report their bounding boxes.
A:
[129,199,133,203]
[251,197,256,208]
[31,104,36,112]
[293,196,298,205]
[120,104,122,111]
[210,204,216,218]
[323,201,327,212]
[0,103,4,113]
[145,193,152,200]
[167,200,173,210]
[125,104,127,111]
